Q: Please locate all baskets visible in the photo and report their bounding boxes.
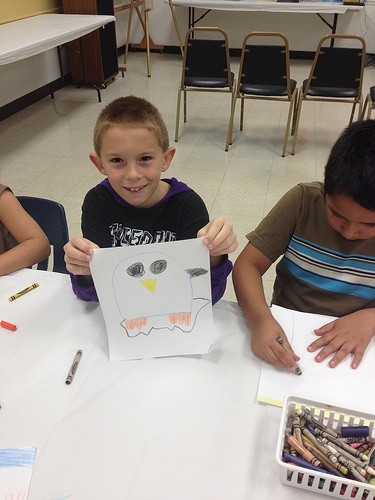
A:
[276,394,375,500]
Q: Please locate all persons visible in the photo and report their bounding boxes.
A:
[0,183,52,276]
[232,118,375,372]
[63,94,239,305]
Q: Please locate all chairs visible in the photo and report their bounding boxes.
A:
[15,196,70,274]
[175,27,375,158]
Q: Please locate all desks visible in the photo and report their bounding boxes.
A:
[164,0,365,48]
[0,13,116,103]
[0,267,344,500]
